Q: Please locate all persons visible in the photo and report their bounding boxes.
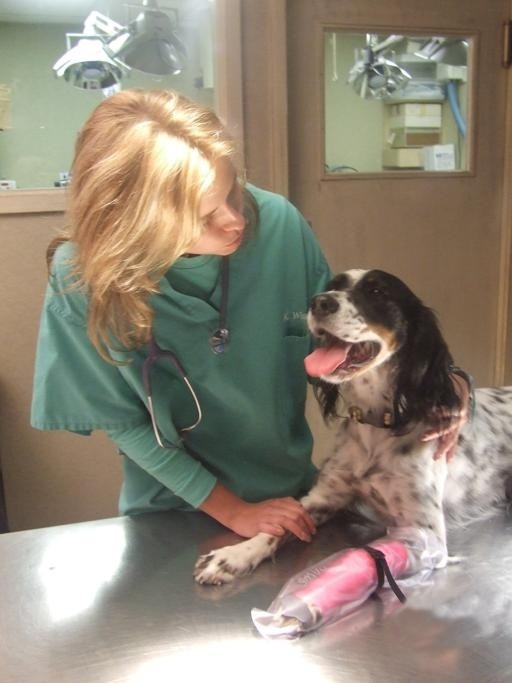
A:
[30,87,469,543]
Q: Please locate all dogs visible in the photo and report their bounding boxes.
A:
[191,267,512,642]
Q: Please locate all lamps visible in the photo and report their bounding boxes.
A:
[346,28,469,100]
[53,0,190,92]
